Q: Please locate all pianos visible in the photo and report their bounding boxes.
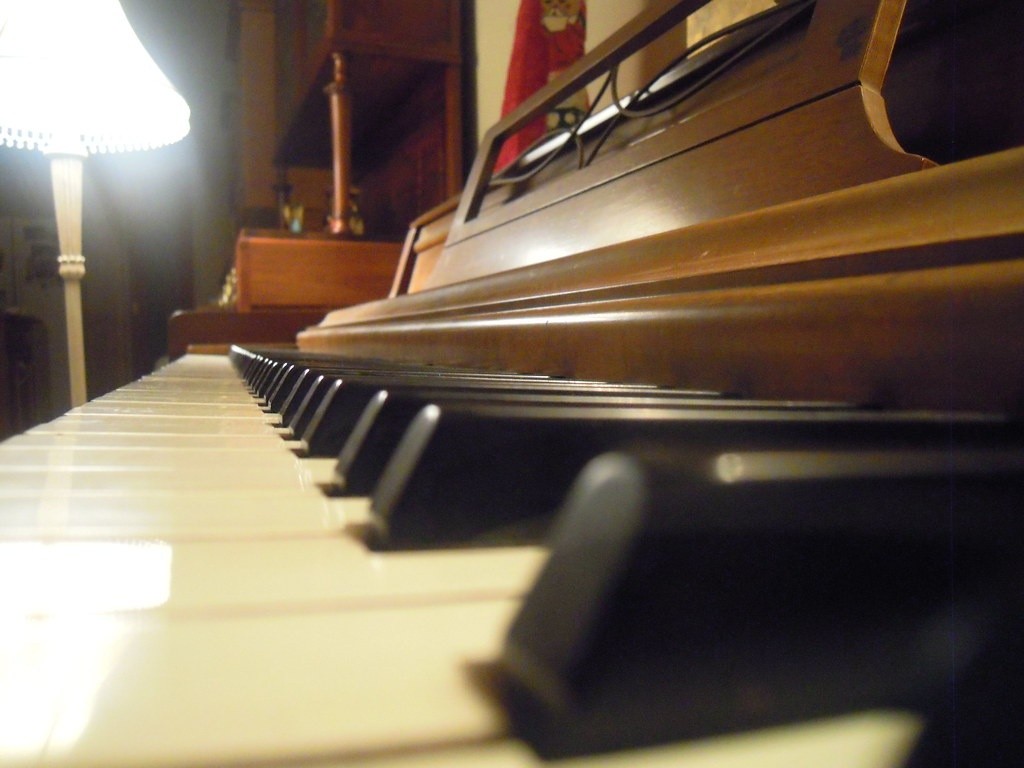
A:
[0,1,1023,768]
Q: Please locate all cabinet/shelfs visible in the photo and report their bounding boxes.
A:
[163,0,472,357]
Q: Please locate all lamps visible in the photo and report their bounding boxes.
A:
[1,0,193,410]
